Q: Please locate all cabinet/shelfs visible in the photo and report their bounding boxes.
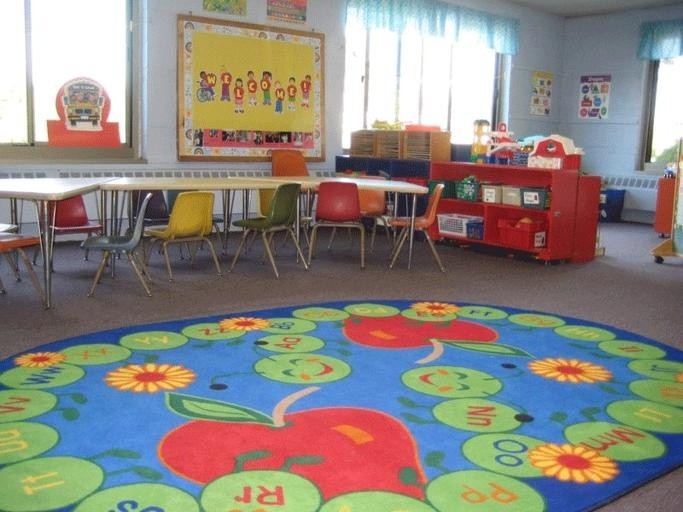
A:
[351,130,451,160]
[335,155,430,242]
[567,175,601,265]
[654,177,675,233]
[430,160,579,265]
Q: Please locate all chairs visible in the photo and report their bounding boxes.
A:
[132,191,169,226]
[33,194,109,272]
[328,176,389,254]
[0,231,47,306]
[81,191,153,298]
[143,191,222,282]
[0,224,18,270]
[167,190,225,250]
[247,189,277,258]
[271,149,310,176]
[307,182,366,269]
[389,183,446,271]
[230,182,309,279]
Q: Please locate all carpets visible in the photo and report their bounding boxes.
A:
[1,300,683,512]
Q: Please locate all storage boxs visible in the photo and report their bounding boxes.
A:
[430,176,550,210]
[497,219,546,249]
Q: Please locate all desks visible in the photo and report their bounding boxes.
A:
[102,176,430,270]
[0,176,118,285]
